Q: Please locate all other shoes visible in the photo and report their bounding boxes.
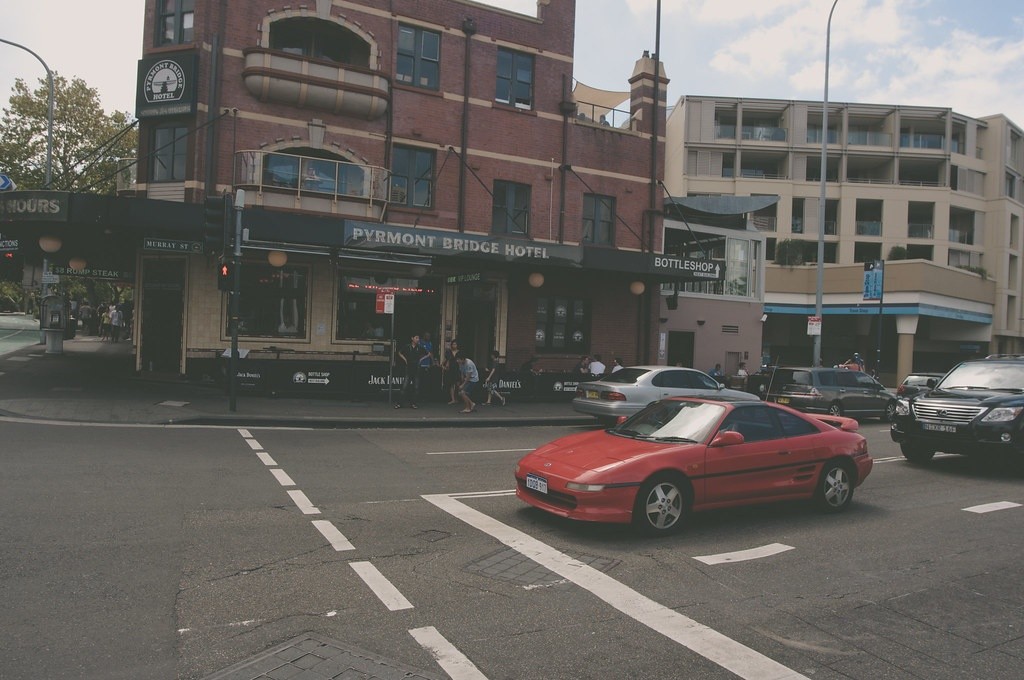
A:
[459,409,471,413]
[501,397,505,406]
[471,403,475,411]
[394,404,401,409]
[409,404,418,409]
[481,402,491,406]
[448,400,455,405]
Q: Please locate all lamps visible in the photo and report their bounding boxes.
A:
[38,232,64,253]
[268,250,288,267]
[69,256,88,270]
[528,272,545,288]
[630,280,646,295]
[666,280,679,310]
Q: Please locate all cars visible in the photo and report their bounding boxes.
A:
[513,394,874,538]
[572,364,761,429]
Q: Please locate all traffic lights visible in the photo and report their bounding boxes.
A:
[0,249,16,261]
[218,263,234,292]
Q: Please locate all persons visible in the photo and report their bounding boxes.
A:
[738,362,749,376]
[79,302,124,344]
[612,358,624,373]
[573,355,606,377]
[708,364,724,377]
[304,164,323,192]
[418,332,436,374]
[441,340,480,413]
[482,351,506,407]
[579,112,585,120]
[843,353,865,371]
[599,115,610,126]
[394,333,431,410]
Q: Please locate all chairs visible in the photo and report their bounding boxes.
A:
[572,373,609,392]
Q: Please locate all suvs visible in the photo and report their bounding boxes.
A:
[890,353,1024,471]
[765,365,898,425]
[896,372,946,401]
